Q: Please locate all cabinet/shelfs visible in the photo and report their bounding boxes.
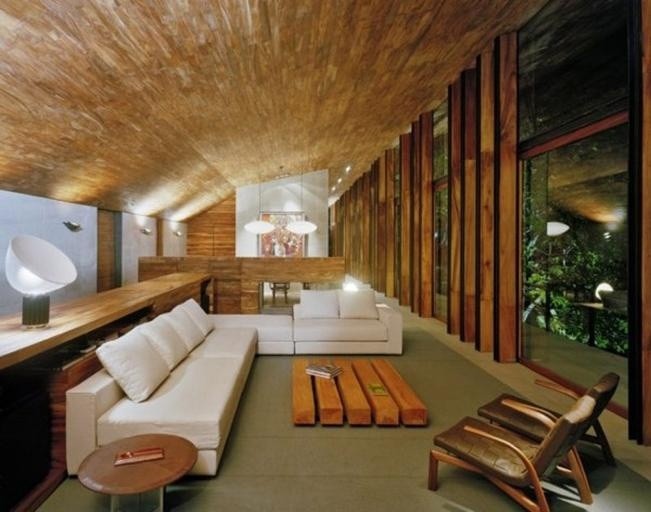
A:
[1,269,217,511]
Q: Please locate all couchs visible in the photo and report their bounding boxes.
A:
[65,327,257,477]
[293,299,404,356]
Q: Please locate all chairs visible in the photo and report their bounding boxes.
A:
[426,395,606,512]
[477,373,628,502]
[269,280,292,306]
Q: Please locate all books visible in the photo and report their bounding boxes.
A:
[304,362,343,379]
[367,383,389,396]
[23,315,148,372]
[114,445,165,466]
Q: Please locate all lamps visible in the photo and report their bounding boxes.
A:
[142,227,152,234]
[245,172,276,236]
[63,219,82,233]
[5,237,78,330]
[287,167,319,236]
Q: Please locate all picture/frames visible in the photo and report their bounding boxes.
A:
[256,209,310,257]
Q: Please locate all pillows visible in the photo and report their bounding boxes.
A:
[97,332,170,403]
[137,318,189,371]
[164,309,205,351]
[340,289,378,320]
[180,298,215,334]
[301,289,340,320]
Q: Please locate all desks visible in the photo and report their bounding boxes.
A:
[77,434,200,511]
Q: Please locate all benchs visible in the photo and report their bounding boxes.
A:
[207,313,294,356]
